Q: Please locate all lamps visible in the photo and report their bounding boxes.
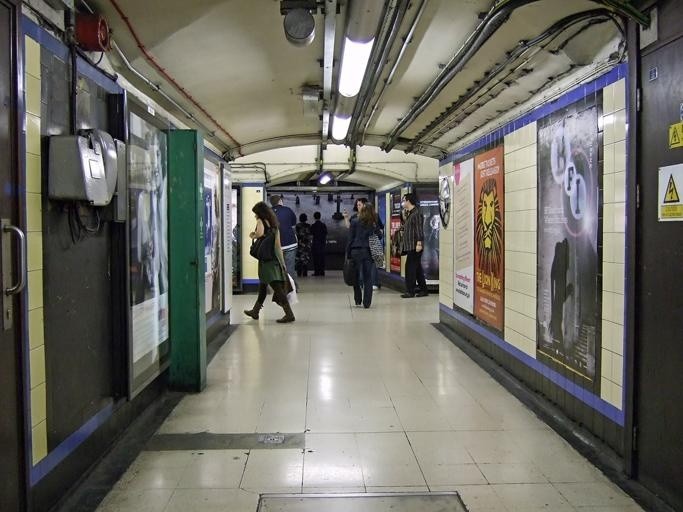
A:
[330,0,386,145]
[318,172,331,185]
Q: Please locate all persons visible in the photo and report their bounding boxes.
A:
[269,195,299,294]
[244,202,295,323]
[310,212,327,276]
[400,193,429,298]
[135,129,169,299]
[347,202,384,308]
[295,213,310,277]
[343,198,382,290]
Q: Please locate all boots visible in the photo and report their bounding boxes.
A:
[277,303,295,322]
[245,302,263,319]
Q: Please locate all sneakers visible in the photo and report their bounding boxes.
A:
[401,293,413,297]
[416,292,428,296]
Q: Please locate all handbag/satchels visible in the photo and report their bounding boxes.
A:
[369,235,384,268]
[250,232,272,260]
[343,263,356,285]
